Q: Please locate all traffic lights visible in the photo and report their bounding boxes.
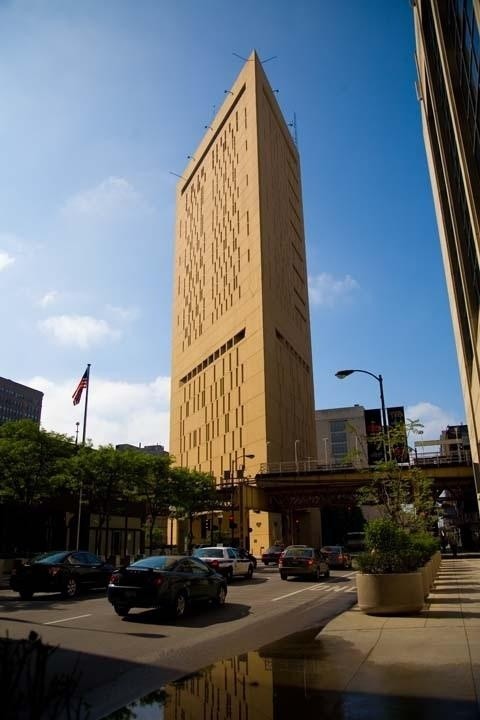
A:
[205,519,211,530]
[229,515,234,528]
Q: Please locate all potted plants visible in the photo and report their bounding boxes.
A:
[342,413,459,616]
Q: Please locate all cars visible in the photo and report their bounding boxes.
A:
[106,555,228,624]
[192,546,257,584]
[9,551,118,601]
[318,545,353,569]
[261,545,286,565]
[278,545,331,581]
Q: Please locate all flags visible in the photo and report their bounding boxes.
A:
[72,364,90,406]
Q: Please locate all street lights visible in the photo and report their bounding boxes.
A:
[231,454,254,547]
[263,426,464,472]
[335,369,391,464]
